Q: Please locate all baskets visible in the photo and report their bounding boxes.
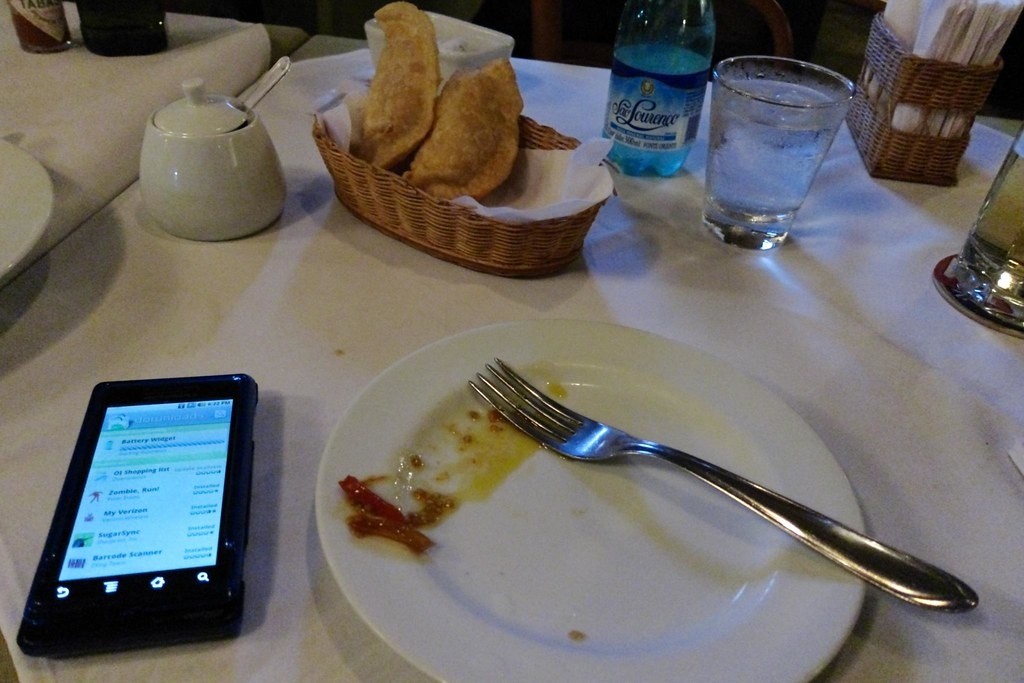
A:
[312,72,621,279]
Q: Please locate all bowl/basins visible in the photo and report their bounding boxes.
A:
[365,10,515,88]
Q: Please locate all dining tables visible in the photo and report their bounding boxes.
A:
[0,1,310,288]
[0,36,1024,681]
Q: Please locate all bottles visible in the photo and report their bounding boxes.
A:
[8,0,72,54]
[601,0,715,178]
[77,0,168,58]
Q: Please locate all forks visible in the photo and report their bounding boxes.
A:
[469,356,979,614]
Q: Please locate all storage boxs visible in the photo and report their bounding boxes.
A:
[842,14,1003,187]
[311,90,620,280]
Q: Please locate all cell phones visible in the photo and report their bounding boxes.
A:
[17,372,258,659]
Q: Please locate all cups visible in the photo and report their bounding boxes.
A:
[954,118,1024,331]
[700,56,856,251]
[139,77,287,242]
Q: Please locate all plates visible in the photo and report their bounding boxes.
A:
[314,319,868,683]
[0,138,53,277]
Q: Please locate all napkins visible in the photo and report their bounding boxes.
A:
[0,50,1024,683]
[0,1,274,279]
